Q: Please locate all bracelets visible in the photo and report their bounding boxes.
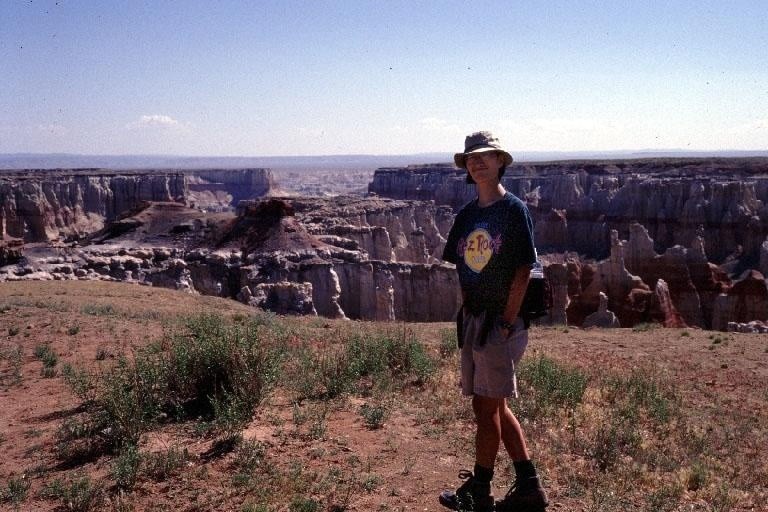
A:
[498,318,512,330]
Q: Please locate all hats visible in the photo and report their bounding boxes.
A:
[454,130,513,170]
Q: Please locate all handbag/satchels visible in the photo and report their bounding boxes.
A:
[517,276,553,320]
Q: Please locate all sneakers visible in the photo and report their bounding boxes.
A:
[438,475,492,511]
[495,475,549,512]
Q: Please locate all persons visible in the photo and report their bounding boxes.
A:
[439,132,550,512]
